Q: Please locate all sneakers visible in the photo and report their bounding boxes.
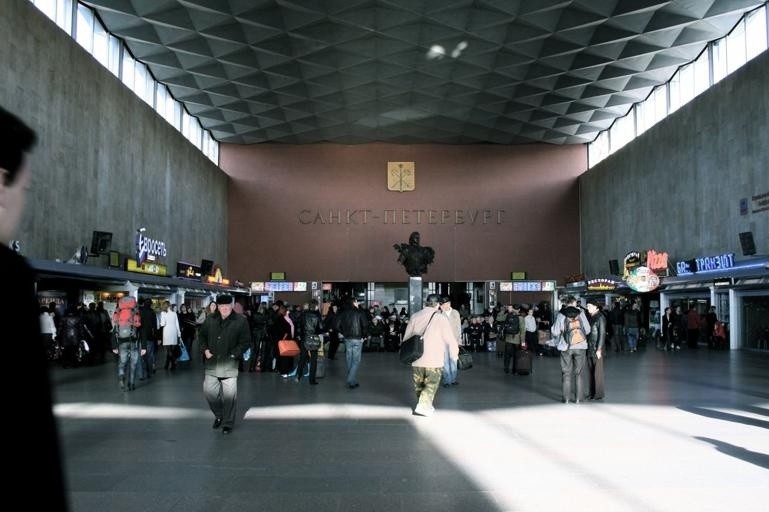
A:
[349,383,360,389]
[452,382,459,385]
[444,383,450,389]
[118,375,135,393]
[310,381,318,385]
[414,404,435,416]
[504,368,510,374]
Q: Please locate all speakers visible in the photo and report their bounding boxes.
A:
[739,232,756,256]
[201,260,213,275]
[91,231,113,255]
[609,260,619,274]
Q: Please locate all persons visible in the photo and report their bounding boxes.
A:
[113,296,196,391]
[397,232,435,276]
[203,294,252,435]
[368,305,408,348]
[551,296,606,404]
[404,294,460,417]
[439,298,461,387]
[0,107,69,511]
[296,298,368,389]
[599,302,644,352]
[199,301,296,378]
[460,302,537,375]
[662,305,717,351]
[41,302,110,368]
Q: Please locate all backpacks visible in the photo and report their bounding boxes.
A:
[504,311,521,336]
[561,315,586,346]
[114,297,141,339]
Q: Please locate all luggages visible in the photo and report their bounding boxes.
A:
[516,349,532,376]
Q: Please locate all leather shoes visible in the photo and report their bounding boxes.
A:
[562,396,603,404]
[223,427,231,435]
[212,417,223,429]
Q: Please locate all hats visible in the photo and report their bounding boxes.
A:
[216,295,232,304]
[427,294,442,303]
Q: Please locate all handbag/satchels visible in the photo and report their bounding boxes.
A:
[278,340,301,357]
[399,335,424,364]
[457,352,473,371]
[304,335,321,350]
[176,344,190,363]
